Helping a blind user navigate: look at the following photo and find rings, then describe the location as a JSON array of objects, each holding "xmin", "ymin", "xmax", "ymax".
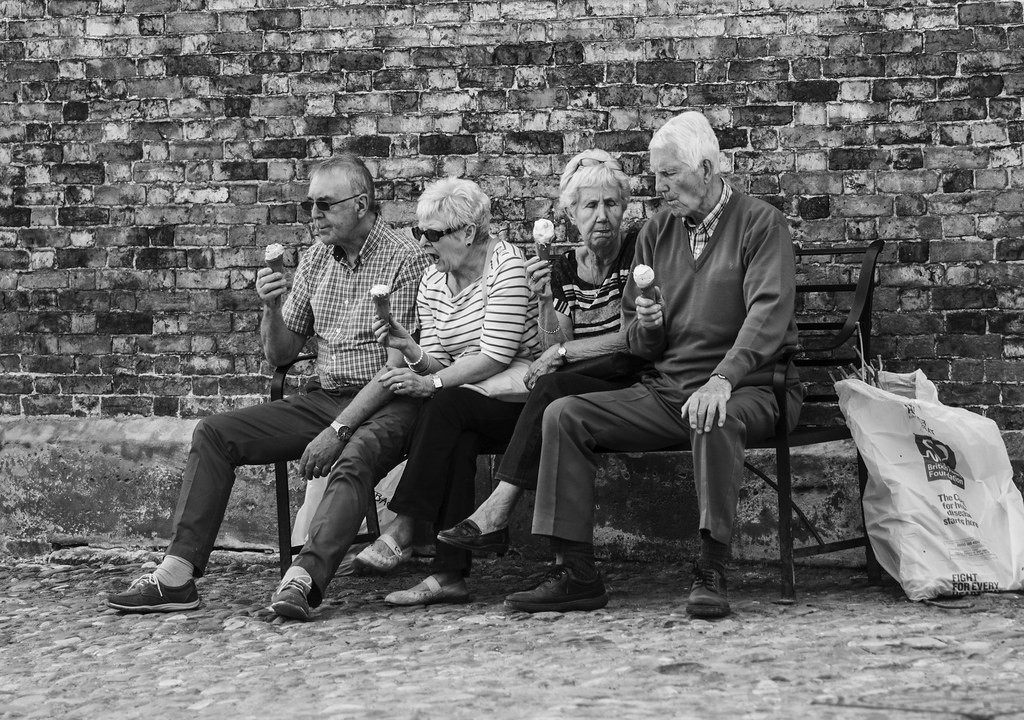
[
  {"xmin": 530, "ymin": 276, "xmax": 538, "ymax": 281},
  {"xmin": 315, "ymin": 466, "xmax": 321, "ymax": 470},
  {"xmin": 397, "ymin": 383, "xmax": 402, "ymax": 390}
]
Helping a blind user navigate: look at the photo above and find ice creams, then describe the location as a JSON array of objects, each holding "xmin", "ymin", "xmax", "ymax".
[
  {"xmin": 265, "ymin": 242, "xmax": 285, "ymax": 305},
  {"xmin": 533, "ymin": 219, "xmax": 554, "ymax": 293},
  {"xmin": 369, "ymin": 285, "xmax": 390, "ymax": 348},
  {"xmin": 633, "ymin": 263, "xmax": 655, "ymax": 305}
]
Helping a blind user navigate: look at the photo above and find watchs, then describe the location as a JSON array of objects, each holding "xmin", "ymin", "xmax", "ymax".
[
  {"xmin": 430, "ymin": 372, "xmax": 443, "ymax": 391},
  {"xmin": 330, "ymin": 421, "xmax": 353, "ymax": 442},
  {"xmin": 558, "ymin": 340, "xmax": 569, "ymax": 366}
]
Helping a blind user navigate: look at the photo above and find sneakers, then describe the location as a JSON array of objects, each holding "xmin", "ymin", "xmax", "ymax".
[
  {"xmin": 271, "ymin": 575, "xmax": 312, "ymax": 618},
  {"xmin": 107, "ymin": 574, "xmax": 200, "ymax": 611},
  {"xmin": 506, "ymin": 564, "xmax": 608, "ymax": 611},
  {"xmin": 687, "ymin": 558, "xmax": 729, "ymax": 615}
]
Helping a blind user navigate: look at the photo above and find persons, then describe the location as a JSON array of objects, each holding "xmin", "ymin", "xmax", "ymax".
[
  {"xmin": 436, "ymin": 148, "xmax": 643, "ymax": 576},
  {"xmin": 352, "ymin": 178, "xmax": 542, "ymax": 606},
  {"xmin": 504, "ymin": 111, "xmax": 809, "ymax": 617},
  {"xmin": 105, "ymin": 155, "xmax": 429, "ymax": 618}
]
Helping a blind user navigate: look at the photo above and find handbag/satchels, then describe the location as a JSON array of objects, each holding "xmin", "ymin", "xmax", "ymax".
[
  {"xmin": 834, "ymin": 368, "xmax": 1024, "ymax": 600},
  {"xmin": 458, "ymin": 357, "xmax": 533, "ymax": 401},
  {"xmin": 290, "ymin": 459, "xmax": 407, "ymax": 549},
  {"xmin": 556, "ymin": 351, "xmax": 643, "ymax": 378}
]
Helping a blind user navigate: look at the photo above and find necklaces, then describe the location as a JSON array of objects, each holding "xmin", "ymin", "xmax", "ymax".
[{"xmin": 589, "ymin": 258, "xmax": 606, "ymax": 296}]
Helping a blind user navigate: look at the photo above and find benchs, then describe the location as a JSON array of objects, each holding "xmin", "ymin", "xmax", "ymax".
[{"xmin": 270, "ymin": 238, "xmax": 884, "ymax": 604}]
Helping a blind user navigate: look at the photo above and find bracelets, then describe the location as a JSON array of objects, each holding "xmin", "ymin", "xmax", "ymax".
[
  {"xmin": 537, "ymin": 317, "xmax": 561, "ymax": 333},
  {"xmin": 403, "ymin": 345, "xmax": 424, "ymax": 366},
  {"xmin": 406, "ymin": 356, "xmax": 432, "ymax": 375},
  {"xmin": 717, "ymin": 373, "xmax": 727, "ymax": 379}
]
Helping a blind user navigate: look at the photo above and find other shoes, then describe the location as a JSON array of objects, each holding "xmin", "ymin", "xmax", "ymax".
[
  {"xmin": 356, "ymin": 536, "xmax": 413, "ymax": 571},
  {"xmin": 437, "ymin": 518, "xmax": 510, "ymax": 552},
  {"xmin": 385, "ymin": 575, "xmax": 469, "ymax": 606}
]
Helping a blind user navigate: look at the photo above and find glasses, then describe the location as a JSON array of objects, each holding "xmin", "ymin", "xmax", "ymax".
[
  {"xmin": 412, "ymin": 224, "xmax": 467, "ymax": 242},
  {"xmin": 575, "ymin": 158, "xmax": 624, "ymax": 173},
  {"xmin": 300, "ymin": 195, "xmax": 359, "ymax": 211}
]
